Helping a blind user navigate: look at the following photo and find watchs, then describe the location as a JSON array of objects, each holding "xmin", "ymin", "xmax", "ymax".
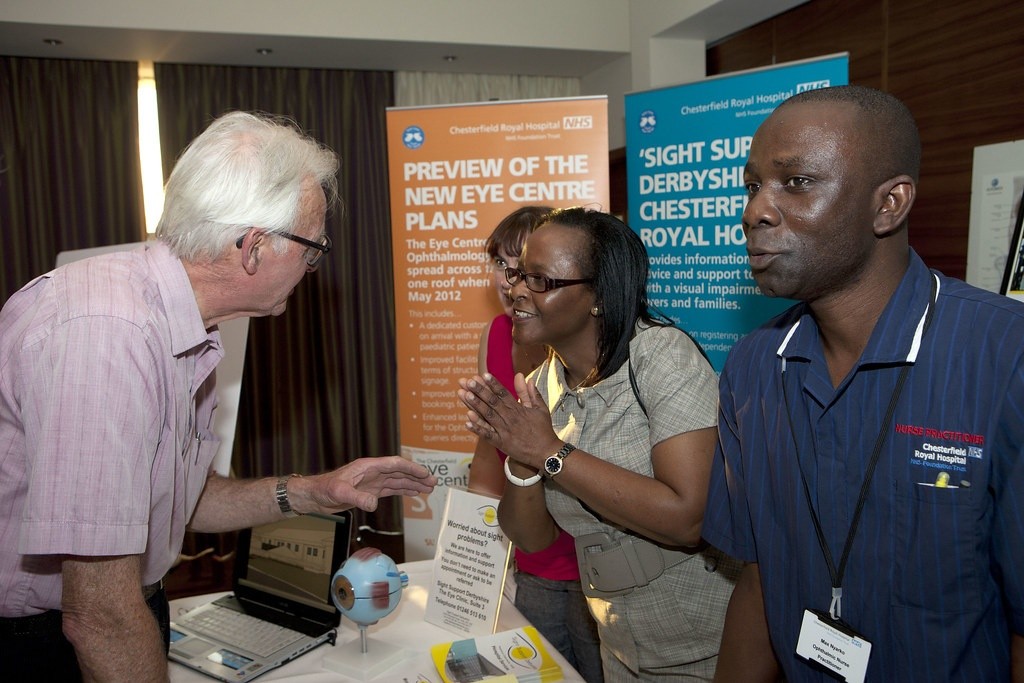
[{"xmin": 543, "ymin": 443, "xmax": 576, "ymax": 481}]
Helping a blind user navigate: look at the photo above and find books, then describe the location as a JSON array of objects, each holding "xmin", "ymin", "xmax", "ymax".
[{"xmin": 431, "ymin": 626, "xmax": 565, "ymax": 682}]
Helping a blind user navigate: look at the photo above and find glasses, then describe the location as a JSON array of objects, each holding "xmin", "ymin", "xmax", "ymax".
[
  {"xmin": 504, "ymin": 266, "xmax": 595, "ymax": 293},
  {"xmin": 236, "ymin": 229, "xmax": 332, "ymax": 267}
]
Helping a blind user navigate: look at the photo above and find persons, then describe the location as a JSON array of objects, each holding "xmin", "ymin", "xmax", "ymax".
[
  {"xmin": 0, "ymin": 110, "xmax": 436, "ymax": 683},
  {"xmin": 459, "ymin": 208, "xmax": 747, "ymax": 683},
  {"xmin": 701, "ymin": 85, "xmax": 1024, "ymax": 683},
  {"xmin": 469, "ymin": 206, "xmax": 604, "ymax": 683}
]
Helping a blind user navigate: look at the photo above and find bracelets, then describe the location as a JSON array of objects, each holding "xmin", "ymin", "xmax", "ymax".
[
  {"xmin": 276, "ymin": 473, "xmax": 302, "ymax": 519},
  {"xmin": 504, "ymin": 456, "xmax": 543, "ymax": 487}
]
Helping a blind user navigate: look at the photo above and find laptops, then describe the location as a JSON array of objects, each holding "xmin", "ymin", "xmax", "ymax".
[{"xmin": 165, "ymin": 508, "xmax": 353, "ymax": 683}]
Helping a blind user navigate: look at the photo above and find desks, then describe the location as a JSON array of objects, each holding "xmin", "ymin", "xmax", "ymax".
[{"xmin": 167, "ymin": 560, "xmax": 588, "ymax": 683}]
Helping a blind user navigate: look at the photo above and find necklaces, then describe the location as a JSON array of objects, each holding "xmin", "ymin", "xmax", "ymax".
[{"xmin": 560, "ymin": 365, "xmax": 595, "ymax": 412}]
